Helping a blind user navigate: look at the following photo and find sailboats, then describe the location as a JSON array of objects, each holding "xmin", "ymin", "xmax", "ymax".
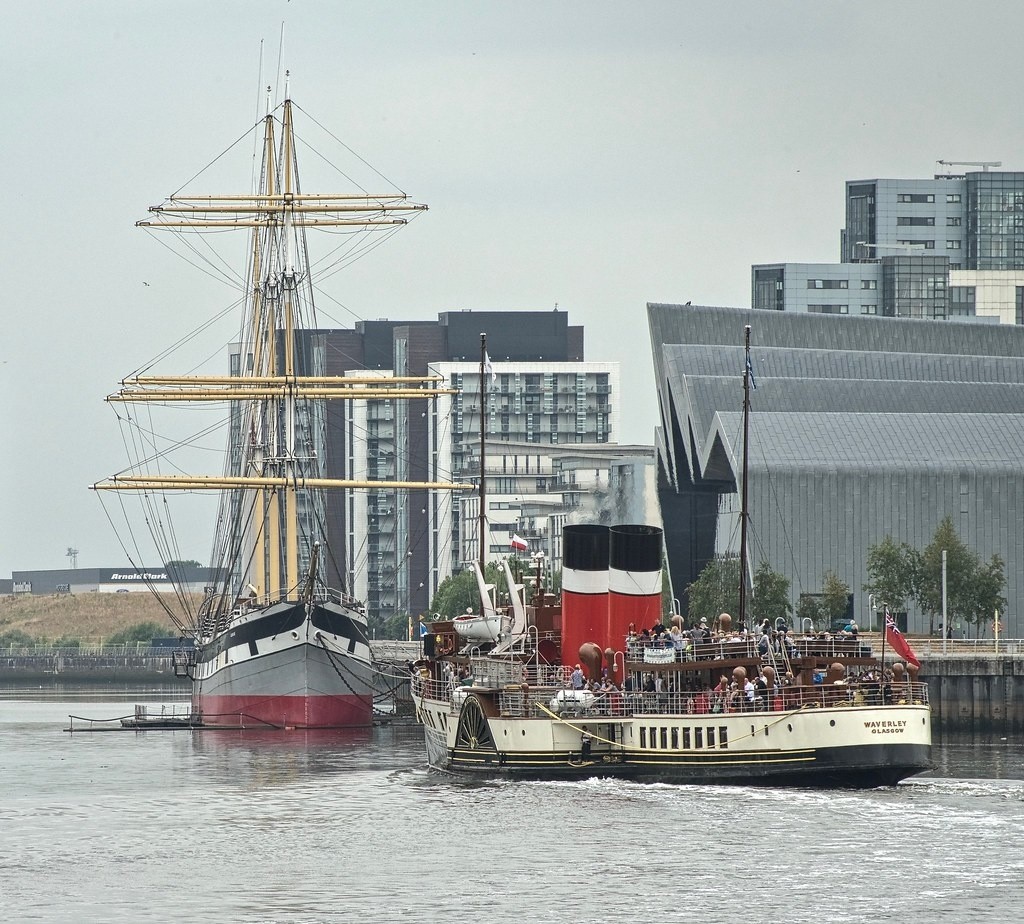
[
  {"xmin": 409, "ymin": 325, "xmax": 933, "ymax": 790},
  {"xmin": 87, "ymin": 21, "xmax": 480, "ymax": 732}
]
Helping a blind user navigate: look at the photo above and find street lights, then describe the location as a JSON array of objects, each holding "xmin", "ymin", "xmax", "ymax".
[
  {"xmin": 519, "ymin": 568, "xmax": 523, "ymax": 606},
  {"xmin": 802, "ymin": 617, "xmax": 814, "ymax": 637},
  {"xmin": 669, "ymin": 598, "xmax": 681, "ymax": 632},
  {"xmin": 869, "ymin": 594, "xmax": 877, "ymax": 631},
  {"xmin": 530, "ymin": 550, "xmax": 545, "ymax": 593}
]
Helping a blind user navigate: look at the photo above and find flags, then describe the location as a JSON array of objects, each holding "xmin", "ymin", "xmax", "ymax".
[{"xmin": 883, "ymin": 607, "xmax": 922, "ymax": 669}]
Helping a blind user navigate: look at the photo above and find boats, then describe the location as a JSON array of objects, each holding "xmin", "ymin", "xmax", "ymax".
[{"xmin": 452, "ymin": 614, "xmax": 512, "ymax": 643}]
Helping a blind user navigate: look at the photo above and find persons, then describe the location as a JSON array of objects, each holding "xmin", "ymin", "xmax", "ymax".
[
  {"xmin": 405, "ymin": 611, "xmax": 895, "ymax": 715},
  {"xmin": 581, "ymin": 725, "xmax": 593, "ymax": 764},
  {"xmin": 512, "ymin": 533, "xmax": 528, "ymax": 550}
]
